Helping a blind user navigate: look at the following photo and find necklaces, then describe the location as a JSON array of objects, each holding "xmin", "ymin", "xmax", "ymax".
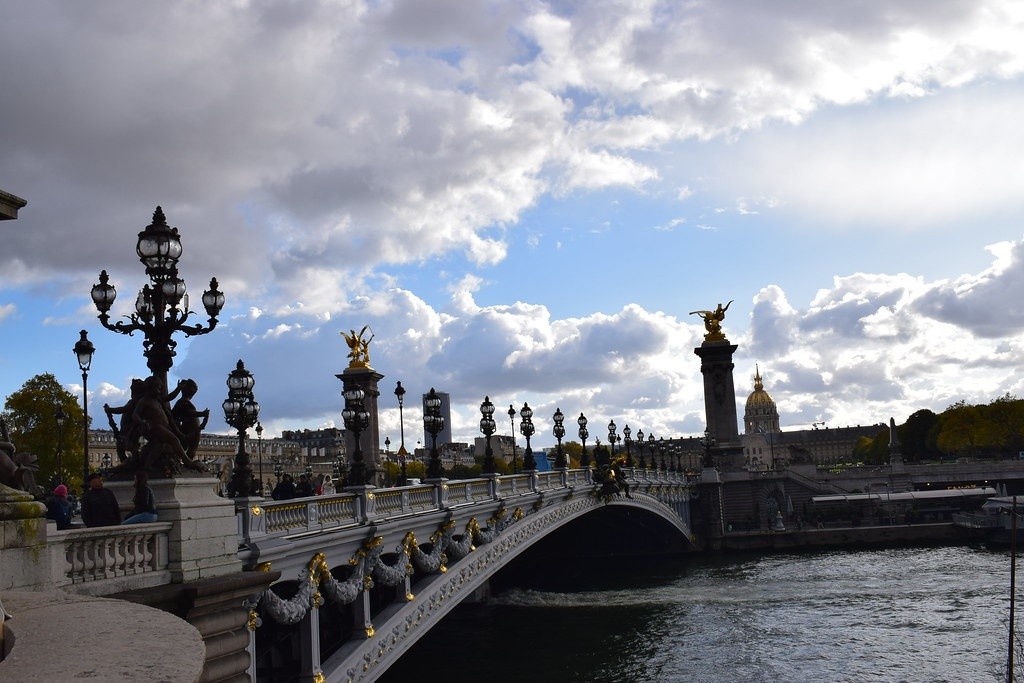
[{"xmin": 321, "ymin": 474, "xmax": 336, "ymax": 496}]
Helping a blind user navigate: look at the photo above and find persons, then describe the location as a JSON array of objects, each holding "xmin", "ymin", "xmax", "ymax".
[
  {"xmin": 378, "ymin": 472, "xmax": 401, "ymax": 488},
  {"xmin": 81, "ymin": 472, "xmax": 121, "ymax": 527},
  {"xmin": 816, "ymin": 515, "xmax": 824, "ymax": 529},
  {"xmin": 132, "ymin": 471, "xmax": 155, "ymax": 507},
  {"xmin": 251, "ymin": 474, "xmax": 315, "ymax": 501},
  {"xmin": 53, "ymin": 485, "xmax": 73, "ymax": 531},
  {"xmin": 599, "ymin": 464, "xmax": 634, "ymax": 499}
]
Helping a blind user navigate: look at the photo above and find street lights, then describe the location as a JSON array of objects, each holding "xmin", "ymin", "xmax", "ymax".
[
  {"xmin": 616, "ymin": 434, "xmax": 621, "ymax": 458},
  {"xmin": 520, "ymin": 401, "xmax": 538, "ymax": 471},
  {"xmin": 221, "ymin": 358, "xmax": 265, "ymax": 499},
  {"xmin": 394, "ymin": 381, "xmax": 407, "ymax": 454},
  {"xmin": 70, "ymin": 330, "xmax": 95, "ymax": 497},
  {"xmin": 422, "ymin": 386, "xmax": 446, "ymax": 479},
  {"xmin": 342, "ymin": 377, "xmax": 372, "ymax": 485},
  {"xmin": 607, "ymin": 420, "xmax": 618, "ymax": 458},
  {"xmin": 274, "ymin": 461, "xmax": 284, "ymax": 485},
  {"xmin": 668, "ymin": 437, "xmax": 676, "ymax": 471},
  {"xmin": 479, "ymin": 395, "xmax": 498, "ymax": 474},
  {"xmin": 417, "ymin": 438, "xmax": 423, "ymax": 462},
  {"xmin": 255, "ymin": 421, "xmax": 264, "ymax": 497},
  {"xmin": 697, "ymin": 426, "xmax": 716, "ymax": 469},
  {"xmin": 385, "ymin": 437, "xmax": 391, "ymax": 485},
  {"xmin": 636, "ymin": 428, "xmax": 647, "ymax": 468},
  {"xmin": 577, "ymin": 412, "xmax": 591, "ymax": 467},
  {"xmin": 688, "ymin": 450, "xmax": 692, "ymax": 470},
  {"xmin": 90, "ymin": 206, "xmax": 225, "ymax": 475},
  {"xmin": 657, "ymin": 436, "xmax": 668, "ymax": 471},
  {"xmin": 507, "ymin": 404, "xmax": 521, "ymax": 474},
  {"xmin": 674, "ymin": 441, "xmax": 683, "ymax": 473},
  {"xmin": 623, "ymin": 424, "xmax": 633, "ymax": 458},
  {"xmin": 552, "ymin": 407, "xmax": 568, "ymax": 470},
  {"xmin": 647, "ymin": 433, "xmax": 658, "ymax": 470}
]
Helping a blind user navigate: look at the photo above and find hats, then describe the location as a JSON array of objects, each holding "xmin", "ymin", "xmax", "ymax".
[{"xmin": 53, "ymin": 485, "xmax": 67, "ymax": 496}]
